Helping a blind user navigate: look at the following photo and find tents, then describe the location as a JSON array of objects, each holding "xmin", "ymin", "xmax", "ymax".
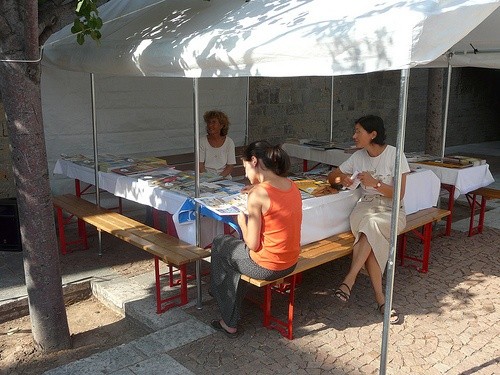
[{"xmin": 41, "ymin": 0, "xmax": 500, "ymax": 375}]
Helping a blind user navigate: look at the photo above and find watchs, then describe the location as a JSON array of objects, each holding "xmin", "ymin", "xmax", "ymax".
[{"xmin": 374, "ymin": 181, "xmax": 381, "ymax": 189}]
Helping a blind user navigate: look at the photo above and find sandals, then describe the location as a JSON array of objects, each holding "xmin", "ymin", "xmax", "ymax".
[
  {"xmin": 378, "ymin": 299, "xmax": 399, "ymax": 324},
  {"xmin": 334, "ymin": 282, "xmax": 353, "ymax": 304}
]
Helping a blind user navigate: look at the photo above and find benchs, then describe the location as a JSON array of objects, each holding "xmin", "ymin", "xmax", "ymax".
[
  {"xmin": 51, "ymin": 192, "xmax": 212, "ymax": 316},
  {"xmin": 239, "ymin": 207, "xmax": 451, "ymax": 339},
  {"xmin": 465, "ymin": 187, "xmax": 500, "ymax": 236}
]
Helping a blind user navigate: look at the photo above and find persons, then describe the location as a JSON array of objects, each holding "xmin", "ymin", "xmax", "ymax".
[
  {"xmin": 208, "ymin": 140, "xmax": 303, "ymax": 339},
  {"xmin": 328, "ymin": 115, "xmax": 411, "ymax": 324},
  {"xmin": 198, "ymin": 111, "xmax": 236, "ymax": 181}
]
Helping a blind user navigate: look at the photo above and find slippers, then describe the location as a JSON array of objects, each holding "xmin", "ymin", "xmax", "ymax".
[{"xmin": 209, "ymin": 318, "xmax": 235, "ymax": 338}]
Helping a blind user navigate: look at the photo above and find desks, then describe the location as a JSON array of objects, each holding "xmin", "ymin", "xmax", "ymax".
[
  {"xmin": 171, "ymin": 165, "xmax": 441, "ymax": 248},
  {"xmin": 52, "ymin": 152, "xmax": 246, "ymax": 251},
  {"xmin": 281, "ymin": 140, "xmax": 495, "ymax": 239}
]
